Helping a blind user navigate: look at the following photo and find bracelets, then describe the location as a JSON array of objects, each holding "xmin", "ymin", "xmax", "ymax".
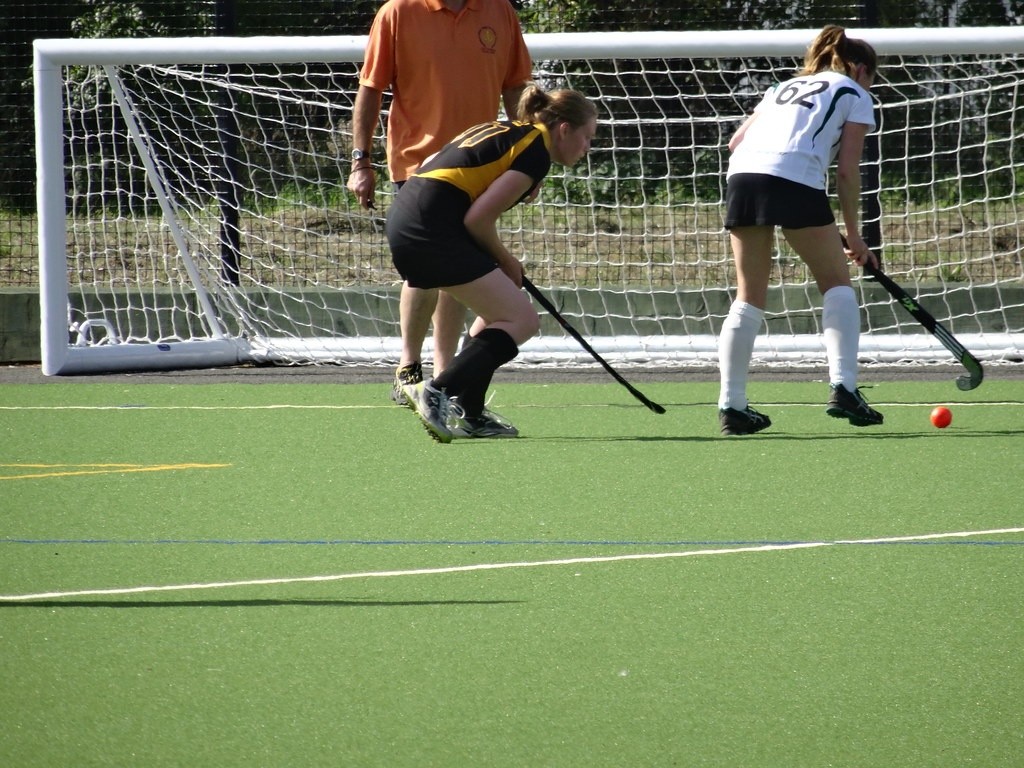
[{"xmin": 349, "ymin": 166, "xmax": 372, "ymax": 174}]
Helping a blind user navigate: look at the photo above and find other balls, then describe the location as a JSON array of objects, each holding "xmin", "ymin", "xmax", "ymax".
[{"xmin": 930, "ymin": 406, "xmax": 953, "ymax": 429}]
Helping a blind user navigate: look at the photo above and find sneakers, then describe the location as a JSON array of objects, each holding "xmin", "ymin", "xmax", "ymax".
[
  {"xmin": 446, "ymin": 396, "xmax": 520, "ymax": 440},
  {"xmin": 719, "ymin": 405, "xmax": 772, "ymax": 436},
  {"xmin": 390, "ymin": 361, "xmax": 424, "ymax": 406},
  {"xmin": 826, "ymin": 383, "xmax": 884, "ymax": 426},
  {"xmin": 398, "ymin": 375, "xmax": 466, "ymax": 444}
]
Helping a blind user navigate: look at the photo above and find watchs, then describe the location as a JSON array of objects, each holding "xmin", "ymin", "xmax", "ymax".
[{"xmin": 351, "ymin": 149, "xmax": 370, "ymax": 160}]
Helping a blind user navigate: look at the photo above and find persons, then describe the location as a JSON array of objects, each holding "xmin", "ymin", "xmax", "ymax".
[
  {"xmin": 346, "ymin": 0, "xmax": 535, "ymax": 404},
  {"xmin": 385, "ymin": 86, "xmax": 597, "ymax": 441},
  {"xmin": 715, "ymin": 24, "xmax": 884, "ymax": 436}
]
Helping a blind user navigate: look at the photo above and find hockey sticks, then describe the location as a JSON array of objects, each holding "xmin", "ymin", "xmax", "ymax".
[
  {"xmin": 521, "ymin": 275, "xmax": 669, "ymax": 416},
  {"xmin": 839, "ymin": 231, "xmax": 987, "ymax": 394}
]
